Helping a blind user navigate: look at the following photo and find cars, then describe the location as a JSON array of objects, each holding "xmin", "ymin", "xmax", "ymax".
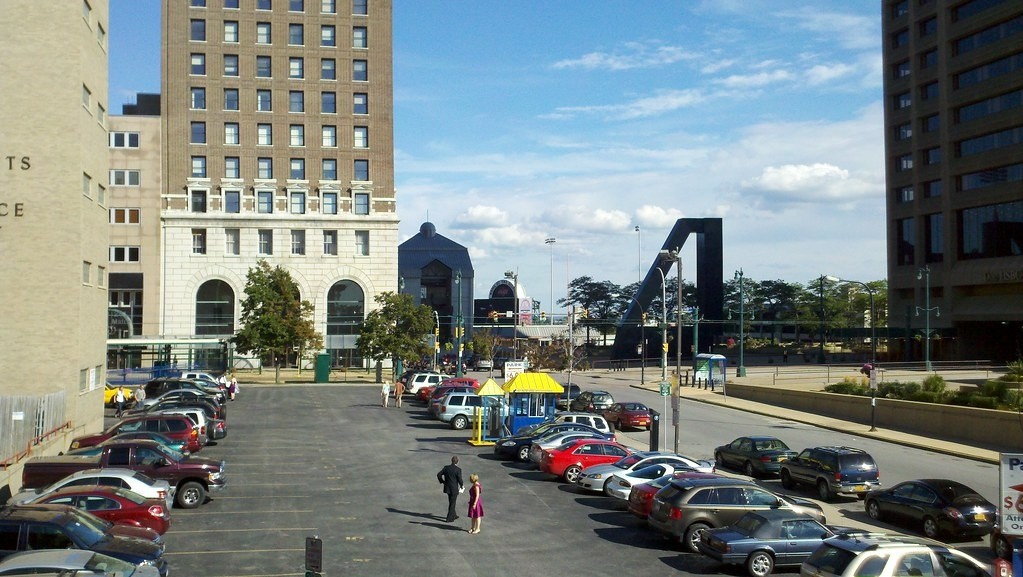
[
  {"xmin": 0, "ymin": 366, "xmax": 230, "ymax": 577},
  {"xmin": 863, "ymin": 478, "xmax": 1000, "ymax": 541},
  {"xmin": 695, "ymin": 509, "xmax": 873, "ymax": 577},
  {"xmin": 574, "ymin": 450, "xmax": 717, "ymax": 498},
  {"xmin": 990, "ymin": 515, "xmax": 1023, "ymax": 565},
  {"xmin": 625, "ymin": 468, "xmax": 762, "ymax": 522},
  {"xmin": 395, "ymin": 359, "xmax": 526, "ymax": 431},
  {"xmin": 605, "ymin": 462, "xmax": 717, "ymax": 504},
  {"xmin": 569, "ymin": 390, "xmax": 616, "ymax": 417},
  {"xmin": 600, "ymin": 401, "xmax": 651, "ymax": 432},
  {"xmin": 799, "ymin": 529, "xmax": 999, "ymax": 577},
  {"xmin": 491, "ymin": 410, "xmax": 653, "ymax": 485},
  {"xmin": 712, "ymin": 434, "xmax": 800, "ymax": 480},
  {"xmin": 554, "ymin": 383, "xmax": 582, "ymax": 410}
]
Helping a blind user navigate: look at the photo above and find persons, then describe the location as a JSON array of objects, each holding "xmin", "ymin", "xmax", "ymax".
[
  {"xmin": 135, "ymin": 385, "xmax": 146, "ymax": 408},
  {"xmin": 231, "ymin": 375, "xmax": 236, "ymax": 401},
  {"xmin": 381, "ymin": 381, "xmax": 390, "ymax": 407},
  {"xmin": 468, "ymin": 474, "xmax": 484, "ymax": 534},
  {"xmin": 394, "ymin": 381, "xmax": 405, "ymax": 407},
  {"xmin": 437, "ymin": 456, "xmax": 464, "ymax": 522},
  {"xmin": 114, "ymin": 386, "xmax": 127, "ymax": 418},
  {"xmin": 860, "ymin": 365, "xmax": 872, "ymax": 378}
]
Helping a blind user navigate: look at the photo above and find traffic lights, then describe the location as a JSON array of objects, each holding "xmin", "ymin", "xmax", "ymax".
[
  {"xmin": 435, "ymin": 327, "xmax": 440, "ymax": 337},
  {"xmin": 541, "ymin": 311, "xmax": 545, "ymax": 321},
  {"xmin": 493, "ymin": 311, "xmax": 498, "ymax": 322}
]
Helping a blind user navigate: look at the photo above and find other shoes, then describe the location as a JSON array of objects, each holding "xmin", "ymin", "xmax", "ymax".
[
  {"xmin": 446, "ymin": 519, "xmax": 454, "ymax": 522},
  {"xmin": 472, "ymin": 529, "xmax": 480, "ymax": 533},
  {"xmin": 454, "ymin": 516, "xmax": 459, "ymax": 519},
  {"xmin": 468, "ymin": 528, "xmax": 475, "ymax": 533}
]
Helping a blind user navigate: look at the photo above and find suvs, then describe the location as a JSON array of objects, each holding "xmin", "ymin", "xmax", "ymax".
[
  {"xmin": 778, "ymin": 444, "xmax": 880, "ymax": 503},
  {"xmin": 646, "ymin": 477, "xmax": 827, "ymax": 555}
]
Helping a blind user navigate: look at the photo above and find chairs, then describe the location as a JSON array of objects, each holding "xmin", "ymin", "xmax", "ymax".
[
  {"xmin": 79, "ymin": 501, "xmax": 87, "ymax": 510},
  {"xmin": 781, "ymin": 526, "xmax": 787, "ymax": 537},
  {"xmin": 763, "ymin": 441, "xmax": 772, "ymax": 448},
  {"xmin": 910, "ymin": 558, "xmax": 930, "ymax": 573},
  {"xmin": 731, "ymin": 495, "xmax": 737, "ymax": 504}
]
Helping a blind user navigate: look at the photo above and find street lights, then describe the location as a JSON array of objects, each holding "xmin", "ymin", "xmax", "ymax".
[
  {"xmin": 913, "ymin": 265, "xmax": 933, "ymax": 372},
  {"xmin": 826, "ymin": 276, "xmax": 877, "ymax": 432},
  {"xmin": 665, "ymin": 255, "xmax": 683, "ymax": 384},
  {"xmin": 634, "ymin": 224, "xmax": 642, "ymax": 286},
  {"xmin": 504, "ymin": 270, "xmax": 517, "ymax": 362},
  {"xmin": 610, "ymin": 293, "xmax": 645, "ymax": 385},
  {"xmin": 394, "ymin": 275, "xmax": 407, "ymax": 379},
  {"xmin": 544, "ymin": 237, "xmax": 557, "ymax": 325},
  {"xmin": 454, "ymin": 270, "xmax": 463, "ymax": 378},
  {"xmin": 733, "ymin": 267, "xmax": 746, "ymax": 378}
]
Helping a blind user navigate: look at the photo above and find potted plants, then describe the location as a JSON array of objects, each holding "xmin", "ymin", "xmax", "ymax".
[{"xmin": 340, "ymin": 359, "xmax": 348, "ymax": 372}]
